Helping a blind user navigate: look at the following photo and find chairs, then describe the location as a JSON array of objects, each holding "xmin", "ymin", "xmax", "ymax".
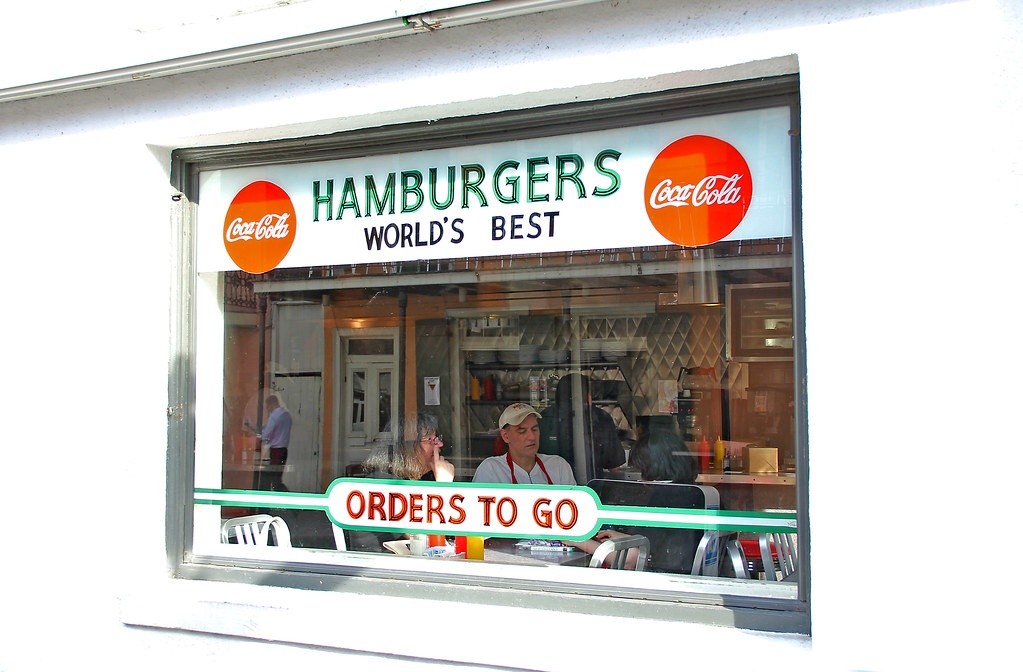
[
  {"xmin": 330, "ymin": 520, "xmax": 350, "ymax": 552},
  {"xmin": 726, "ymin": 539, "xmax": 753, "ymax": 581},
  {"xmin": 222, "ymin": 514, "xmax": 282, "ymax": 546},
  {"xmin": 688, "ymin": 529, "xmax": 742, "ymax": 577},
  {"xmin": 759, "ymin": 529, "xmax": 797, "ymax": 585},
  {"xmin": 589, "ymin": 534, "xmax": 651, "ymax": 573}
]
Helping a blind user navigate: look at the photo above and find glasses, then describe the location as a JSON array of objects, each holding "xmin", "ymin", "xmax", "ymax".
[{"xmin": 417, "ymin": 435, "xmax": 443, "ymax": 445}]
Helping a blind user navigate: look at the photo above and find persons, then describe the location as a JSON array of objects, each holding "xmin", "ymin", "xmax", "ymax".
[
  {"xmin": 536, "ymin": 372, "xmax": 628, "ymax": 482},
  {"xmin": 356, "ymin": 410, "xmax": 456, "ymax": 540},
  {"xmin": 256, "ymin": 394, "xmax": 302, "ymax": 517},
  {"xmin": 470, "ymin": 402, "xmax": 578, "ymax": 486},
  {"xmin": 560, "ymin": 428, "xmax": 700, "ymax": 575}
]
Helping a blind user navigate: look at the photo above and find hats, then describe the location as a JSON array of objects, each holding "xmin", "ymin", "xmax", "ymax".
[{"xmin": 499, "ymin": 402, "xmax": 542, "ymax": 430}]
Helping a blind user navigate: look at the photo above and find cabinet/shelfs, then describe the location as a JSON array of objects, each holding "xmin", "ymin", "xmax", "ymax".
[{"xmin": 454, "ymin": 313, "xmax": 695, "ymax": 479}]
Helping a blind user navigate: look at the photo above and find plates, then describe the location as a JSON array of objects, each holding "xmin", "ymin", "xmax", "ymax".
[{"xmin": 460, "ymin": 334, "xmax": 628, "ymax": 366}]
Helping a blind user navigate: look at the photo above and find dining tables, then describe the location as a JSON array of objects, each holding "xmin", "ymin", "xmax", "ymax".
[{"xmin": 368, "ymin": 537, "xmax": 594, "ymax": 568}]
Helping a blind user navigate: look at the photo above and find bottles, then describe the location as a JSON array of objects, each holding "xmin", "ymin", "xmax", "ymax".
[
  {"xmin": 471, "ymin": 375, "xmax": 480, "ymax": 400},
  {"xmin": 713, "ymin": 435, "xmax": 726, "ymax": 472},
  {"xmin": 483, "ymin": 373, "xmax": 494, "ymax": 401},
  {"xmin": 698, "ymin": 434, "xmax": 711, "ymax": 471},
  {"xmin": 722, "ymin": 452, "xmax": 742, "ymax": 473},
  {"xmin": 496, "ymin": 379, "xmax": 502, "ymax": 400},
  {"xmin": 235, "ymin": 438, "xmax": 270, "ymax": 463}
]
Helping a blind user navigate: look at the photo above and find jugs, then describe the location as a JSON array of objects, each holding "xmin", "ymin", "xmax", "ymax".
[{"xmin": 677, "ymin": 365, "xmax": 714, "ymax": 390}]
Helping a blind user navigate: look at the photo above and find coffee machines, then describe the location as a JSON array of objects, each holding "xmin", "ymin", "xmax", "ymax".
[{"xmin": 677, "ymin": 388, "xmax": 726, "ymax": 442}]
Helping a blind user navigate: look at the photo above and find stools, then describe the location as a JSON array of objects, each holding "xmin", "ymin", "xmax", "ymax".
[{"xmin": 733, "ymin": 539, "xmax": 794, "ymax": 581}]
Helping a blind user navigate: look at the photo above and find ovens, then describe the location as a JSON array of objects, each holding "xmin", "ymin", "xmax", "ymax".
[{"xmin": 724, "ymin": 284, "xmax": 794, "ymax": 361}]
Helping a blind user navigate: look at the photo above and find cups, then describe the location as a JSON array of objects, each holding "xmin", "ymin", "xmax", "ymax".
[
  {"xmin": 467, "ymin": 536, "xmax": 484, "ymax": 560},
  {"xmin": 428, "ymin": 534, "xmax": 444, "ymax": 548},
  {"xmin": 409, "ymin": 534, "xmax": 427, "ymax": 556},
  {"xmin": 454, "ymin": 535, "xmax": 467, "ymax": 560}
]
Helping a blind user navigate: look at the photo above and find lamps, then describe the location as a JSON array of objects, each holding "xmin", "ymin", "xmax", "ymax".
[{"xmin": 678, "ymin": 249, "xmax": 721, "ymax": 307}]
